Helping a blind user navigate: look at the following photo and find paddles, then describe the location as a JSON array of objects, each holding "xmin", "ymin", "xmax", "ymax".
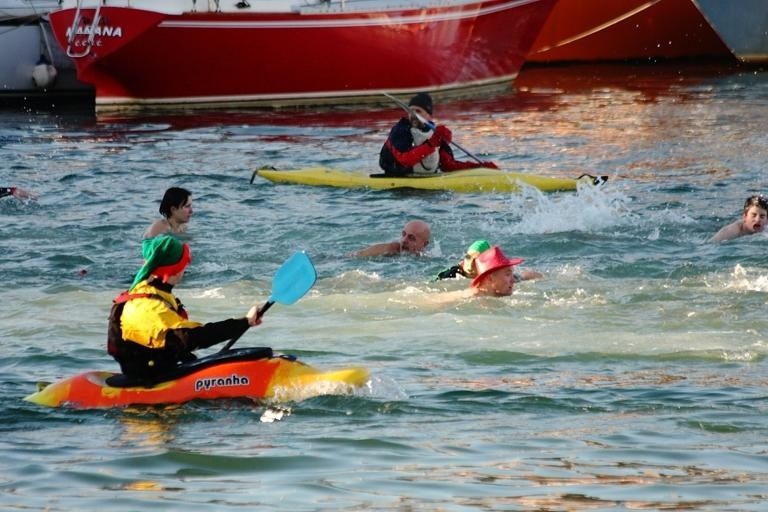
[
  {"xmin": 217, "ymin": 251, "xmax": 316, "ymax": 352},
  {"xmin": 383, "ymin": 92, "xmax": 485, "ymax": 164}
]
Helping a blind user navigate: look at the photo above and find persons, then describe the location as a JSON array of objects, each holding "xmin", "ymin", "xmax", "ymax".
[
  {"xmin": 0, "ymin": 187, "xmax": 38, "ymax": 205},
  {"xmin": 355, "ymin": 220, "xmax": 431, "ymax": 257},
  {"xmin": 430, "ymin": 240, "xmax": 542, "ymax": 282},
  {"xmin": 143, "ymin": 187, "xmax": 193, "ymax": 239},
  {"xmin": 470, "ymin": 245, "xmax": 525, "ymax": 297},
  {"xmin": 707, "ymin": 196, "xmax": 768, "ymax": 243},
  {"xmin": 107, "ymin": 233, "xmax": 263, "ymax": 389},
  {"xmin": 379, "ymin": 93, "xmax": 497, "ymax": 178}
]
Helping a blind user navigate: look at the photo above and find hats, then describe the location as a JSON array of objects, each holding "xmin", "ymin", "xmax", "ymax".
[
  {"xmin": 128, "ymin": 233, "xmax": 188, "ymax": 291},
  {"xmin": 408, "ymin": 93, "xmax": 432, "ymax": 115},
  {"xmin": 468, "ymin": 240, "xmax": 523, "ymax": 287}
]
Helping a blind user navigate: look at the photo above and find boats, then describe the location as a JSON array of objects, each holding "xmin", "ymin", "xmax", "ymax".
[
  {"xmin": 252, "ymin": 158, "xmax": 614, "ymax": 200},
  {"xmin": 0, "ymin": 0, "xmax": 104, "ymax": 100},
  {"xmin": 22, "ymin": 347, "xmax": 370, "ymax": 418},
  {"xmin": 519, "ymin": 0, "xmax": 768, "ymax": 64},
  {"xmin": 43, "ymin": 0, "xmax": 561, "ymax": 124}
]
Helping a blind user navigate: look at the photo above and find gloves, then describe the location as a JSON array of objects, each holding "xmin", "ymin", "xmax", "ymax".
[{"xmin": 429, "ymin": 124, "xmax": 451, "ymax": 146}]
